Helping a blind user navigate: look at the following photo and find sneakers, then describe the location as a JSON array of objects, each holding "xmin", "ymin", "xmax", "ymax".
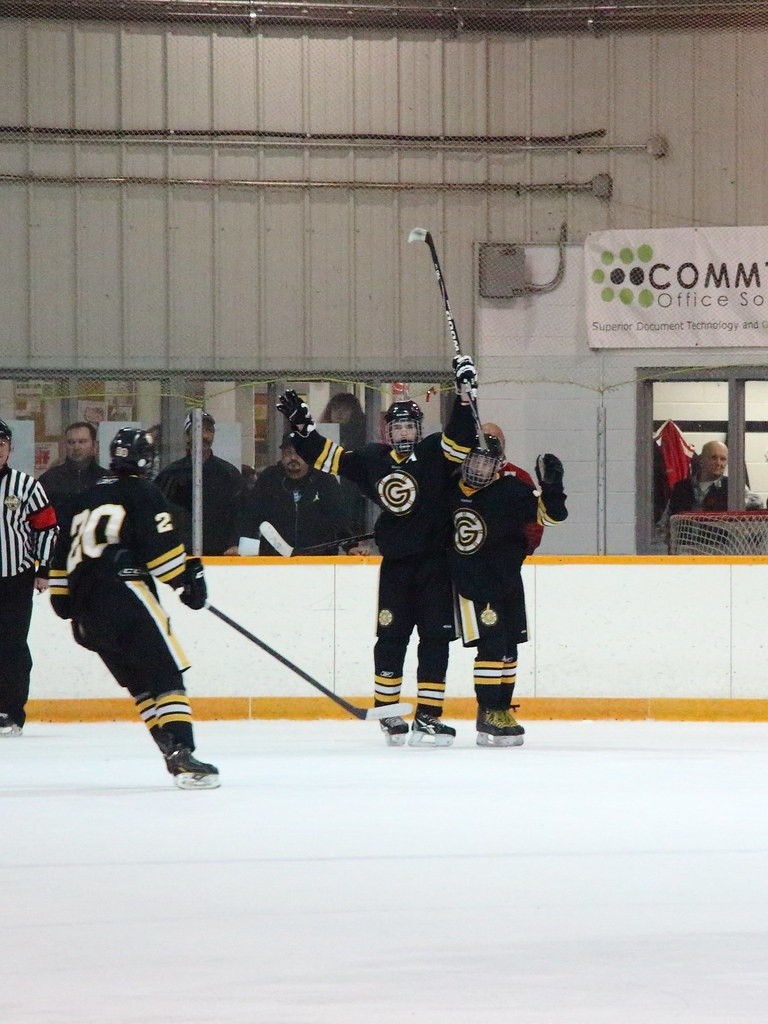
[
  {"xmin": 158, "ymin": 734, "xmax": 225, "ymax": 790},
  {"xmin": 408, "ymin": 705, "xmax": 456, "ymax": 746},
  {"xmin": 381, "ymin": 717, "xmax": 409, "ymax": 747},
  {"xmin": 476, "ymin": 704, "xmax": 524, "ymax": 748},
  {"xmin": 0, "ymin": 711, "xmax": 22, "ymax": 737}
]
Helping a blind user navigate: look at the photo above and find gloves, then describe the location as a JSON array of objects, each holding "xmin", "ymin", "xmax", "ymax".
[
  {"xmin": 48, "ymin": 569, "xmax": 73, "ymax": 619},
  {"xmin": 536, "ymin": 452, "xmax": 564, "ymax": 489},
  {"xmin": 169, "ymin": 557, "xmax": 207, "ymax": 610},
  {"xmin": 452, "ymin": 356, "xmax": 478, "ymax": 399},
  {"xmin": 276, "ymin": 391, "xmax": 315, "ymax": 437}
]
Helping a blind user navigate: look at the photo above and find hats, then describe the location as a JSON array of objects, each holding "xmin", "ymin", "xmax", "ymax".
[
  {"xmin": 280, "ymin": 431, "xmax": 294, "ymax": 450},
  {"xmin": 183, "ymin": 410, "xmax": 215, "ymax": 433}
]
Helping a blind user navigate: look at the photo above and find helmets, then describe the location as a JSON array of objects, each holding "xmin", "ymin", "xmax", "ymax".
[
  {"xmin": 383, "ymin": 400, "xmax": 423, "ymax": 452},
  {"xmin": 0, "ymin": 419, "xmax": 12, "ymax": 439},
  {"xmin": 461, "ymin": 434, "xmax": 504, "ymax": 489},
  {"xmin": 110, "ymin": 427, "xmax": 160, "ymax": 481}
]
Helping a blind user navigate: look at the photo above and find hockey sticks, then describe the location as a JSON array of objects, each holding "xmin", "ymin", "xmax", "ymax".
[
  {"xmin": 258, "ymin": 520, "xmax": 376, "ymax": 558},
  {"xmin": 176, "ymin": 583, "xmax": 414, "ymax": 722},
  {"xmin": 406, "ymin": 225, "xmax": 491, "ymax": 455}
]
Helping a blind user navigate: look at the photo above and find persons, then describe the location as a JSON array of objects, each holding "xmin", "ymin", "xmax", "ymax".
[
  {"xmin": 670, "ymin": 441, "xmax": 728, "ymax": 544},
  {"xmin": 481, "ymin": 423, "xmax": 544, "ymax": 556},
  {"xmin": 153, "ymin": 410, "xmax": 242, "ymax": 556},
  {"xmin": 317, "ymin": 393, "xmax": 367, "ymax": 542},
  {"xmin": 223, "ymin": 433, "xmax": 371, "ymax": 557},
  {"xmin": 451, "ymin": 434, "xmax": 568, "ymax": 747},
  {"xmin": 38, "ymin": 422, "xmax": 109, "ymax": 511},
  {"xmin": 0, "ymin": 419, "xmax": 60, "ymax": 737},
  {"xmin": 277, "ymin": 354, "xmax": 481, "ymax": 747},
  {"xmin": 49, "ymin": 427, "xmax": 221, "ymax": 790}
]
